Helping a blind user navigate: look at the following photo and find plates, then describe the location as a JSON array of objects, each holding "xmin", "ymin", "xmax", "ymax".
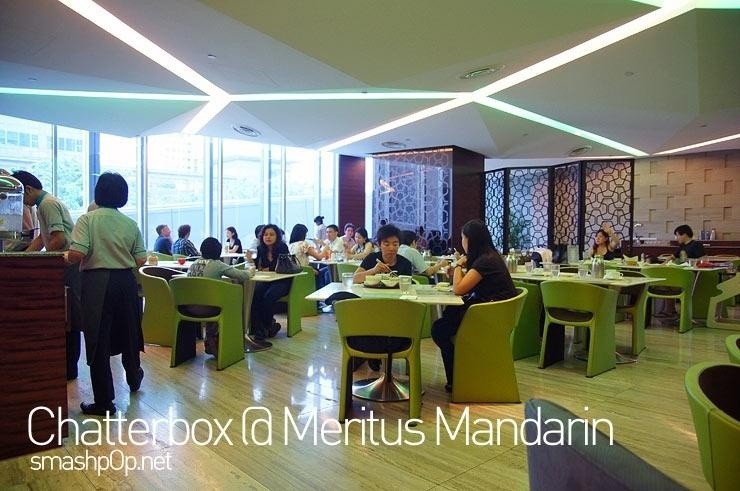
[
  {"xmin": 415, "ymin": 282, "xmax": 451, "ymax": 296},
  {"xmin": 605, "ymin": 276, "xmax": 623, "ymax": 280}
]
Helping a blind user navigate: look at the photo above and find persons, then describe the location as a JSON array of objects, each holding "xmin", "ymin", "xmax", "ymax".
[
  {"xmin": 63, "ymin": 172, "xmax": 147, "ymax": 417},
  {"xmin": 588, "ymin": 222, "xmax": 620, "ymax": 260},
  {"xmin": 431, "ymin": 220, "xmax": 516, "ymax": 393},
  {"xmin": 656, "ymin": 225, "xmax": 707, "ymax": 319},
  {"xmin": 4, "ymin": 172, "xmax": 450, "ymax": 381}
]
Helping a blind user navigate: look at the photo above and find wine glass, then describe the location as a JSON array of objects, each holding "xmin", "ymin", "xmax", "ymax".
[{"xmin": 178, "ymin": 259, "xmax": 186, "ymax": 271}]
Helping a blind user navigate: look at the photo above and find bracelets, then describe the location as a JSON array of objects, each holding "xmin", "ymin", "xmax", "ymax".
[{"xmin": 453, "ymin": 264, "xmax": 463, "ymax": 271}]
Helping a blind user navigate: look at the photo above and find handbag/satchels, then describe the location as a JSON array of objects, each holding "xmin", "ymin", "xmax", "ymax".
[{"xmin": 275, "ymin": 254, "xmax": 303, "ymax": 274}]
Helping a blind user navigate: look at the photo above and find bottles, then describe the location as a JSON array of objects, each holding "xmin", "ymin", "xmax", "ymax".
[
  {"xmin": 149, "ymin": 253, "xmax": 158, "ymax": 266},
  {"xmin": 592, "ymin": 256, "xmax": 604, "ymax": 279},
  {"xmin": 506, "ymin": 253, "xmax": 516, "ymax": 274}
]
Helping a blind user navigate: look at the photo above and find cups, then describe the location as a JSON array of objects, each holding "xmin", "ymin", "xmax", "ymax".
[
  {"xmin": 578, "ymin": 266, "xmax": 588, "ymax": 280},
  {"xmin": 342, "ymin": 273, "xmax": 354, "ymax": 291},
  {"xmin": 398, "ymin": 276, "xmax": 412, "ymax": 295},
  {"xmin": 524, "ymin": 263, "xmax": 533, "ymax": 276},
  {"xmin": 605, "ymin": 270, "xmax": 616, "ymax": 280},
  {"xmin": 551, "ymin": 265, "xmax": 560, "ymax": 279}
]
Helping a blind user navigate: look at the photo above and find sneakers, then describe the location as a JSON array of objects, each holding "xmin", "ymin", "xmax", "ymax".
[
  {"xmin": 129, "ymin": 367, "xmax": 144, "ymax": 391},
  {"xmin": 80, "ymin": 401, "xmax": 117, "ymax": 416}
]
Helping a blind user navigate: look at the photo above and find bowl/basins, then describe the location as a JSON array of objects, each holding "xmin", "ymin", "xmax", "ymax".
[
  {"xmin": 381, "ymin": 280, "xmax": 399, "ymax": 289},
  {"xmin": 365, "ymin": 276, "xmax": 382, "ymax": 286}
]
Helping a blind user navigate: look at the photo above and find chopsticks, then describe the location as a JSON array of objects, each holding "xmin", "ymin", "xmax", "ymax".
[{"xmin": 376, "ymin": 258, "xmax": 398, "ymax": 278}]
